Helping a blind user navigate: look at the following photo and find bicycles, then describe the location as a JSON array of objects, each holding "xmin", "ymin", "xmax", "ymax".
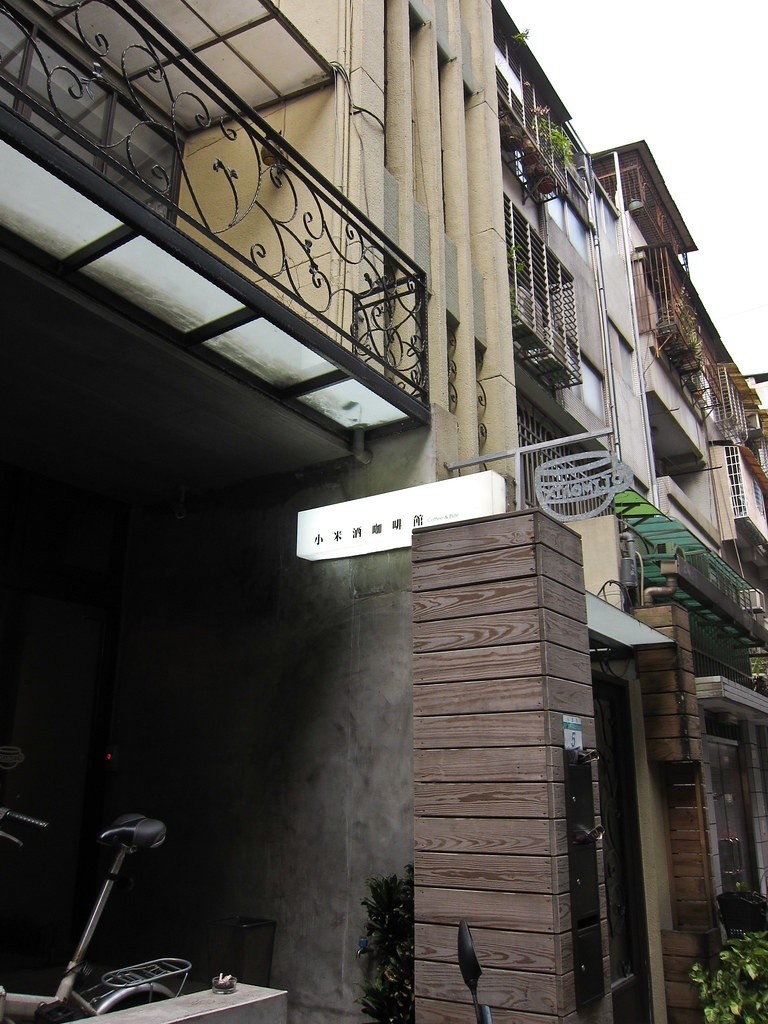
[{"xmin": 0, "ymin": 800, "xmax": 195, "ymax": 1024}]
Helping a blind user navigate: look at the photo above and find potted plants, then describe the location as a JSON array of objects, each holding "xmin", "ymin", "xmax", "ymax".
[
  {"xmin": 500, "ymin": 100, "xmax": 575, "ymax": 194},
  {"xmin": 260, "ymin": 128, "xmax": 282, "ymax": 165}
]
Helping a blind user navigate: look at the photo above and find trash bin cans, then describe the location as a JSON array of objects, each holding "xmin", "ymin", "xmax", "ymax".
[
  {"xmin": 717, "ymin": 892, "xmax": 768, "ymax": 938},
  {"xmin": 205, "ymin": 915, "xmax": 277, "ymax": 987}
]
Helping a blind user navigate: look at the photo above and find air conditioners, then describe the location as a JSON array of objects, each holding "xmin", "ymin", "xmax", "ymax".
[
  {"xmin": 513, "ymin": 286, "xmax": 545, "ymax": 350},
  {"xmin": 745, "ymin": 410, "xmax": 764, "ymax": 439},
  {"xmin": 739, "ymin": 588, "xmax": 765, "ymax": 613}
]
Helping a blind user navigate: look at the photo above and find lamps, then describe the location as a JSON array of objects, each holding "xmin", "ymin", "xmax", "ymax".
[
  {"xmin": 572, "ymin": 748, "xmax": 600, "ymax": 765},
  {"xmin": 579, "ymin": 825, "xmax": 605, "ymax": 844},
  {"xmin": 627, "ymin": 197, "xmax": 645, "ymax": 213}
]
